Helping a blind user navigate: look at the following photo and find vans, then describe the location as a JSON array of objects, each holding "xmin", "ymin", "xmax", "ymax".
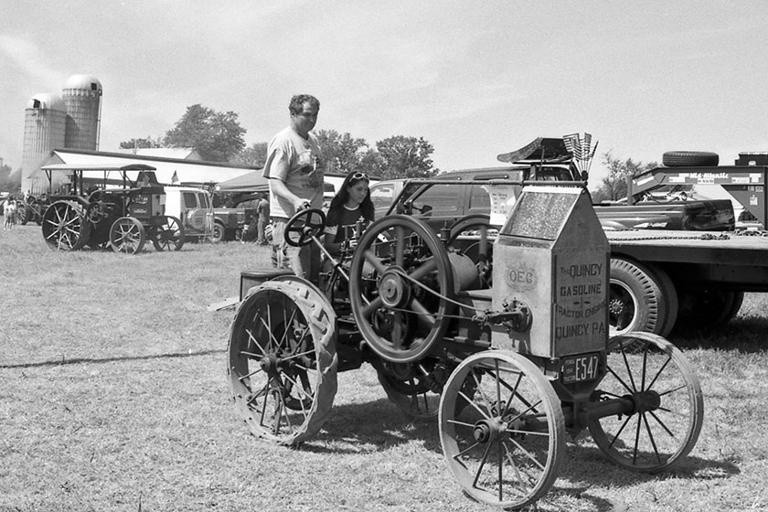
[{"xmin": 162, "ymin": 185, "xmax": 214, "ymax": 244}]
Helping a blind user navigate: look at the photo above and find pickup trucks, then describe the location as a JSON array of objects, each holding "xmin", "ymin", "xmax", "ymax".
[{"xmin": 207, "ymin": 207, "xmax": 257, "ymax": 244}]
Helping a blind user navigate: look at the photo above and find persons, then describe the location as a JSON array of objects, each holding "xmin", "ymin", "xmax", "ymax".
[
  {"xmin": 256, "ymin": 193, "xmax": 269, "ymax": 245},
  {"xmin": 240, "ymin": 220, "xmax": 251, "ymax": 244},
  {"xmin": 262, "ymin": 92, "xmax": 324, "ymax": 280},
  {"xmin": 3, "ymin": 196, "xmax": 19, "ymax": 231},
  {"xmin": 322, "ymin": 168, "xmax": 378, "ymax": 256}
]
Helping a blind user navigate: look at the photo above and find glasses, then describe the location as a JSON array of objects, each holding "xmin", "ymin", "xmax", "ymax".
[{"xmin": 350, "ymin": 172, "xmax": 371, "ymax": 181}]
